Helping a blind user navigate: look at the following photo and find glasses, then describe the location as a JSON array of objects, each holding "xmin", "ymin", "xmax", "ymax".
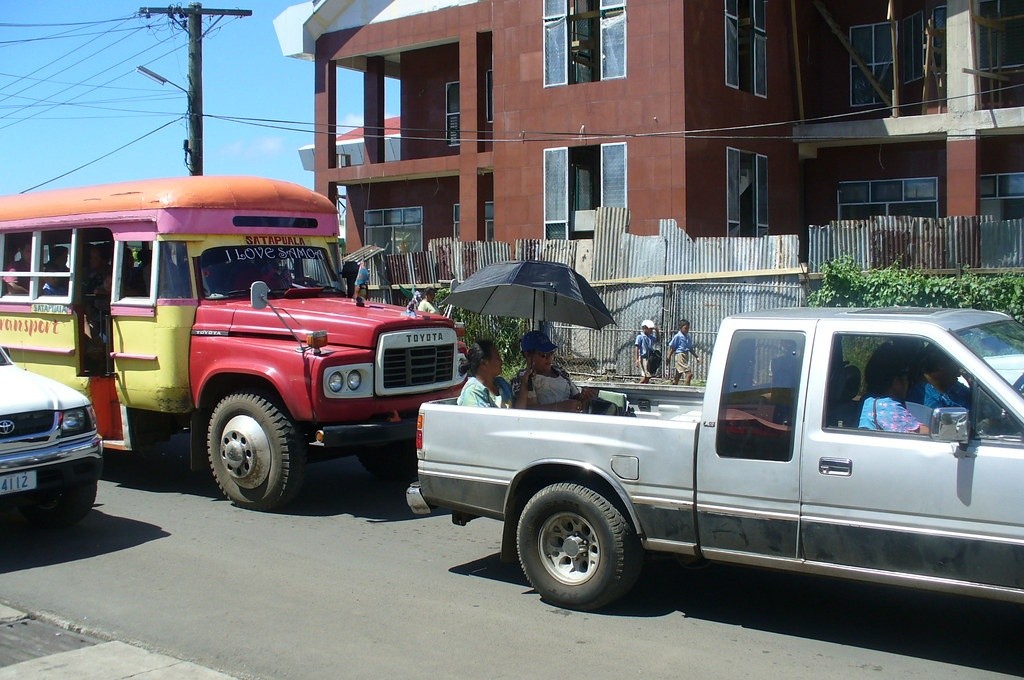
[{"xmin": 536, "ymin": 351, "xmax": 555, "ymax": 358}]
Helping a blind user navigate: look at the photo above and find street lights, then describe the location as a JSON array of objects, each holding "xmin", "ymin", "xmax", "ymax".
[{"xmin": 136, "ymin": 65, "xmax": 205, "ymax": 176}]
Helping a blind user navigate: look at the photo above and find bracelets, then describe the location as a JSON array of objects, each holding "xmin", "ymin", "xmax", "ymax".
[{"xmin": 555, "ymin": 401, "xmax": 557, "ymax": 411}]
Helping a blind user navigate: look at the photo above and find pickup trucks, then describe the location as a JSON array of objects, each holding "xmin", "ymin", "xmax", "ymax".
[{"xmin": 405, "ymin": 307, "xmax": 1024, "ymax": 613}]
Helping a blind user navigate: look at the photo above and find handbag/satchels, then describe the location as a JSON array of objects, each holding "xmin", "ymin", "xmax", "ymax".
[{"xmin": 646, "ymin": 350, "xmax": 662, "ymax": 373}]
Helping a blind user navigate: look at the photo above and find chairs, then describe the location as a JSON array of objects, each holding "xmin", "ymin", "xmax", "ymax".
[
  {"xmin": 829, "ymin": 365, "xmax": 861, "ymax": 400},
  {"xmin": 200, "ymin": 262, "xmax": 229, "ymax": 293}
]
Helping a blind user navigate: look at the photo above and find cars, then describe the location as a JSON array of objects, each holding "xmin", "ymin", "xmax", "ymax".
[{"xmin": 0, "ymin": 345, "xmax": 104, "ymax": 530}]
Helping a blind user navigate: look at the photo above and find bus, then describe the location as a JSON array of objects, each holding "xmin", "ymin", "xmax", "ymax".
[{"xmin": 1, "ymin": 174, "xmax": 470, "ymax": 512}]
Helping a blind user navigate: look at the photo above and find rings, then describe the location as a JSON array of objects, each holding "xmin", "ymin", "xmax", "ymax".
[{"xmin": 576, "ymin": 406, "xmax": 580, "ymax": 410}]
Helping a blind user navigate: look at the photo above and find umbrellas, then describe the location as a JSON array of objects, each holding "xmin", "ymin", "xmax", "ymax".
[{"xmin": 441, "ymin": 260, "xmax": 617, "ymax": 332}]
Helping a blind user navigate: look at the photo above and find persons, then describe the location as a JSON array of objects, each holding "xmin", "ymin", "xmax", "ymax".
[
  {"xmin": 635, "ymin": 319, "xmax": 700, "ymax": 386},
  {"xmin": 2, "ymin": 238, "xmax": 295, "ymax": 378},
  {"xmin": 457, "ymin": 331, "xmax": 599, "ymax": 413},
  {"xmin": 855, "ymin": 342, "xmax": 994, "ymax": 438},
  {"xmin": 418, "ymin": 286, "xmax": 440, "ymax": 315}
]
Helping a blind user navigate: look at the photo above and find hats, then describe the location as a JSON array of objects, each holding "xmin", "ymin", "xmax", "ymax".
[
  {"xmin": 521, "ymin": 331, "xmax": 558, "ymax": 352},
  {"xmin": 642, "ymin": 319, "xmax": 654, "ymax": 328}
]
